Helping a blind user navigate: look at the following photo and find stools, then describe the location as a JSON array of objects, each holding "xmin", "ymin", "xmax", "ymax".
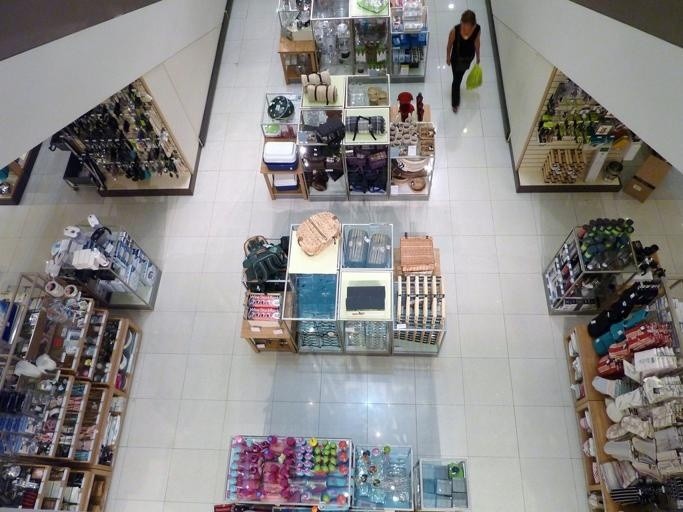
[{"xmin": 63, "ymin": 152, "xmax": 99, "ymax": 191}]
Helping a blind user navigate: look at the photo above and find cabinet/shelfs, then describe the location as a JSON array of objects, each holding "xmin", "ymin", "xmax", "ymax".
[
  {"xmin": 57, "ymin": 222, "xmax": 161, "ymax": 311},
  {"xmin": 260, "ymin": 71, "xmax": 436, "ymax": 202},
  {"xmin": 542, "ymin": 225, "xmax": 681, "ymax": 512},
  {"xmin": 275, "ymin": 0, "xmax": 428, "ymax": 85},
  {"xmin": 1, "ymin": 269, "xmax": 143, "ymax": 510},
  {"xmin": 239, "ymin": 222, "xmax": 447, "ymax": 357}
]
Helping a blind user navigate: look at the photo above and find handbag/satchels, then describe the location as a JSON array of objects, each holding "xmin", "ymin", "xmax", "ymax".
[
  {"xmin": 301, "ymin": 70, "xmax": 331, "ymax": 93},
  {"xmin": 296, "ymin": 213, "xmax": 340, "ymax": 256},
  {"xmin": 348, "ymin": 116, "xmax": 384, "ymax": 134},
  {"xmin": 305, "ymin": 84, "xmax": 337, "ymax": 103},
  {"xmin": 345, "ymin": 147, "xmax": 387, "ymax": 193},
  {"xmin": 317, "ymin": 120, "xmax": 345, "ymax": 142},
  {"xmin": 304, "ymin": 146, "xmax": 344, "ymax": 191}
]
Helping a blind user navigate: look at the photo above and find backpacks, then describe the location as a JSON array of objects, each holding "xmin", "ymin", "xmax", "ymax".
[{"xmin": 243, "ymin": 244, "xmax": 287, "ymax": 283}]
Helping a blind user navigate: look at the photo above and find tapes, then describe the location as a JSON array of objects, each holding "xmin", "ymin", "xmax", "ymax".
[
  {"xmin": 44, "ymin": 281, "xmax": 77, "ymax": 300},
  {"xmin": 390, "ymin": 124, "xmax": 418, "ymax": 147}
]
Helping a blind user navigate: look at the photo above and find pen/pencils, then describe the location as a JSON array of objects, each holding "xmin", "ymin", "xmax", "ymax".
[{"xmin": 56, "ymin": 383, "xmax": 102, "ymax": 461}]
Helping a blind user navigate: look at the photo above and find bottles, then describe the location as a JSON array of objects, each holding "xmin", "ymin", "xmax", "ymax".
[
  {"xmin": 355, "ymin": 40, "xmax": 386, "ymax": 69},
  {"xmin": 622, "ymin": 280, "xmax": 662, "ymax": 305}
]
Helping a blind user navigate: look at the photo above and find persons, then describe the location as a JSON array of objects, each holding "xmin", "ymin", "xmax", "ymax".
[{"xmin": 447, "ymin": 9, "xmax": 481, "ymax": 113}]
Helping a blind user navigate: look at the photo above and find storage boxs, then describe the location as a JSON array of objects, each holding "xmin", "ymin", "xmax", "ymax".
[{"xmin": 624, "ymin": 153, "xmax": 671, "ymax": 204}]
